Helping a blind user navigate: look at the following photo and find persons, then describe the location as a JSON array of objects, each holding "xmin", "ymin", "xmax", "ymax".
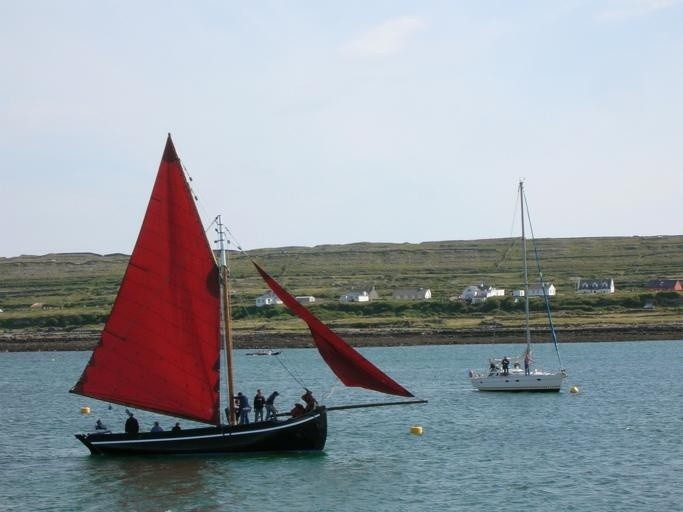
[
  {"xmin": 502, "ymin": 356, "xmax": 509, "ymax": 372},
  {"xmin": 489, "ymin": 359, "xmax": 498, "ymax": 376},
  {"xmin": 266, "ymin": 391, "xmax": 279, "ymax": 421},
  {"xmin": 233, "ymin": 391, "xmax": 251, "ymax": 425},
  {"xmin": 525, "ymin": 354, "xmax": 534, "ymax": 375},
  {"xmin": 292, "ymin": 403, "xmax": 305, "ymax": 417},
  {"xmin": 96, "ymin": 419, "xmax": 107, "ymax": 430},
  {"xmin": 172, "ymin": 422, "xmax": 181, "ymax": 431},
  {"xmin": 254, "ymin": 390, "xmax": 265, "ymax": 423},
  {"xmin": 150, "ymin": 421, "xmax": 163, "ymax": 432},
  {"xmin": 125, "ymin": 413, "xmax": 139, "ymax": 433}
]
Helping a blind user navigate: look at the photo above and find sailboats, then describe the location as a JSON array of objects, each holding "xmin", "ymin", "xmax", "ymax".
[
  {"xmin": 67, "ymin": 131, "xmax": 430, "ymax": 460},
  {"xmin": 468, "ymin": 175, "xmax": 568, "ymax": 392}
]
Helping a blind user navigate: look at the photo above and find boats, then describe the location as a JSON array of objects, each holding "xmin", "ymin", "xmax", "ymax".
[{"xmin": 245, "ymin": 351, "xmax": 281, "ymax": 356}]
